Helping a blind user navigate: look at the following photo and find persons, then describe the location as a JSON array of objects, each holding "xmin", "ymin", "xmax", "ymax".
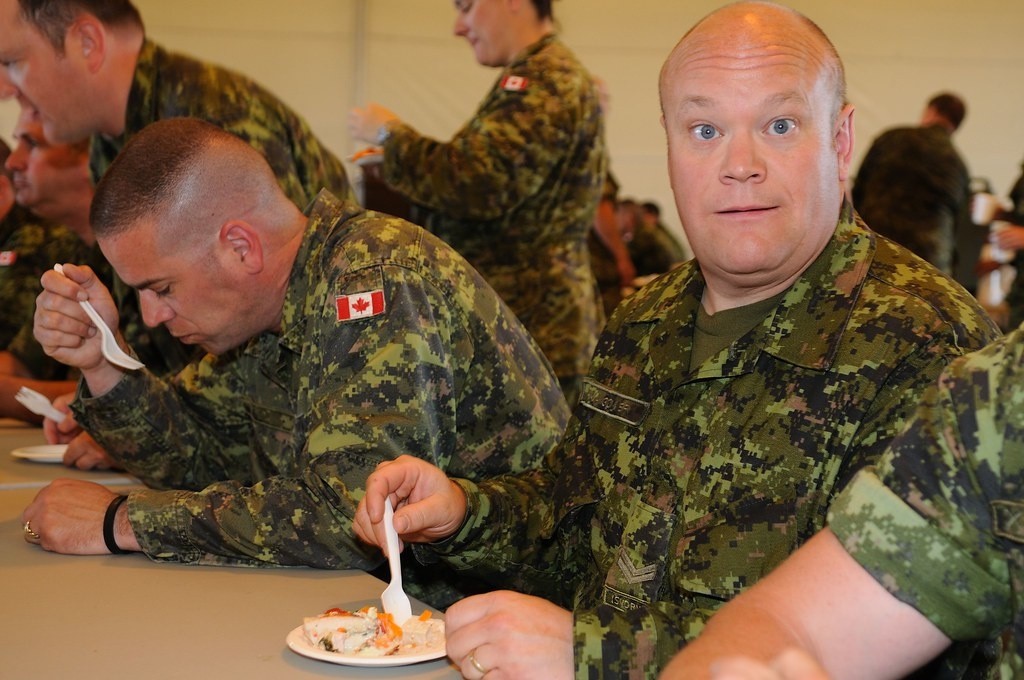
[
  {"xmin": 20, "ymin": 116, "xmax": 572, "ymax": 612},
  {"xmin": 346, "ymin": 0, "xmax": 606, "ymax": 412},
  {"xmin": 991, "ymin": 175, "xmax": 1024, "ymax": 335},
  {"xmin": 352, "ymin": 0, "xmax": 1003, "ymax": 680},
  {"xmin": 0, "ymin": 138, "xmax": 88, "ymax": 350},
  {"xmin": 587, "ymin": 172, "xmax": 685, "ymax": 312},
  {"xmin": 659, "ymin": 323, "xmax": 1023, "ymax": 679},
  {"xmin": 0, "ymin": 116, "xmax": 169, "ymax": 427},
  {"xmin": 850, "ymin": 94, "xmax": 974, "ymax": 277},
  {"xmin": 1, "ymin": 0, "xmax": 359, "ymax": 217}
]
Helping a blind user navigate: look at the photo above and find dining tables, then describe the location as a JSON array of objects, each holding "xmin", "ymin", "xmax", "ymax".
[
  {"xmin": 0, "ymin": 486, "xmax": 468, "ymax": 679},
  {"xmin": 0, "ymin": 413, "xmax": 137, "ymax": 486}
]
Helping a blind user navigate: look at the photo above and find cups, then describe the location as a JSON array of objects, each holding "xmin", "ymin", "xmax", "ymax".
[
  {"xmin": 988, "ymin": 220, "xmax": 1015, "ymax": 263},
  {"xmin": 970, "ymin": 193, "xmax": 997, "ymax": 226}
]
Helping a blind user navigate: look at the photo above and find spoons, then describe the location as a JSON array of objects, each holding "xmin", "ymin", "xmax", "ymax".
[{"xmin": 382, "ymin": 498, "xmax": 412, "ymax": 627}]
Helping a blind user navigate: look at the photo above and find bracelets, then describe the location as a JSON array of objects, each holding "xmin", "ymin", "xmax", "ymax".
[{"xmin": 103, "ymin": 495, "xmax": 128, "ymax": 554}]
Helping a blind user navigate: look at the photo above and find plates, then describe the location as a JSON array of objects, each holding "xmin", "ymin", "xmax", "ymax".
[
  {"xmin": 352, "ymin": 153, "xmax": 385, "ymax": 165},
  {"xmin": 286, "ymin": 614, "xmax": 450, "ymax": 666},
  {"xmin": 10, "ymin": 444, "xmax": 71, "ymax": 462}
]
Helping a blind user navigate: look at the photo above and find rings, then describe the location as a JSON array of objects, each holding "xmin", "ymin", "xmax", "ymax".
[
  {"xmin": 470, "ymin": 649, "xmax": 488, "ymax": 673},
  {"xmin": 24, "ymin": 520, "xmax": 39, "ymax": 539}
]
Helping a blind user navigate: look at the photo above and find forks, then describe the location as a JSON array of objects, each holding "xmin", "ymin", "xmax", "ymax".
[
  {"xmin": 54, "ymin": 263, "xmax": 146, "ymax": 370},
  {"xmin": 14, "ymin": 387, "xmax": 67, "ymax": 422}
]
